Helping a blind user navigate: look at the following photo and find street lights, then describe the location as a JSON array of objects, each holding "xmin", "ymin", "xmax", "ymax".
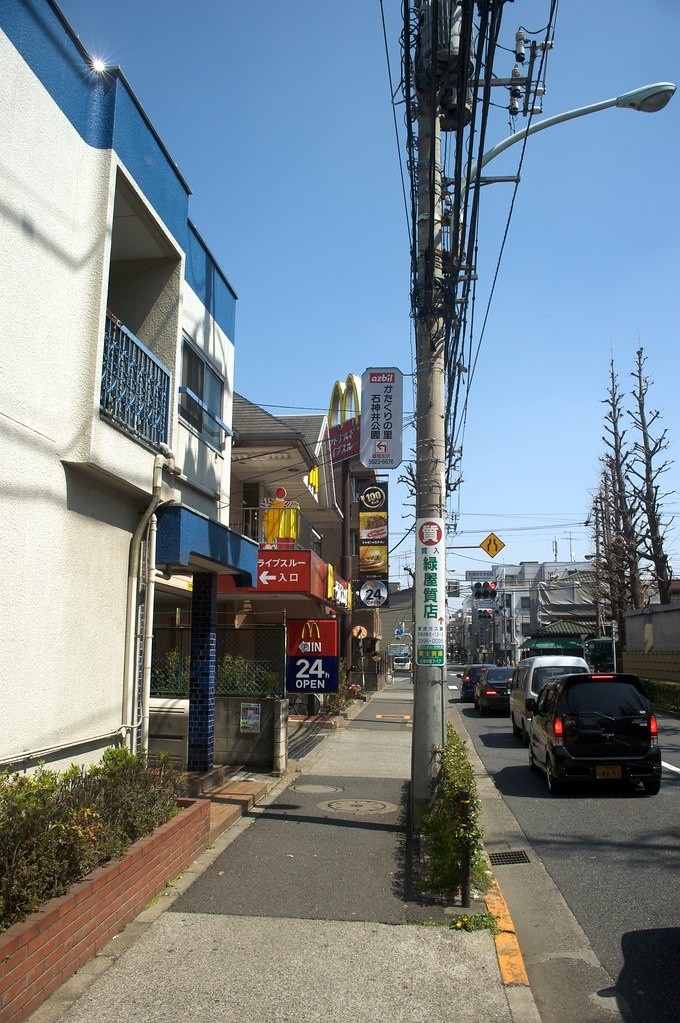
[
  {"xmin": 584, "ymin": 554, "xmax": 617, "ymax": 672},
  {"xmin": 411, "ymin": 82, "xmax": 673, "ymax": 808}
]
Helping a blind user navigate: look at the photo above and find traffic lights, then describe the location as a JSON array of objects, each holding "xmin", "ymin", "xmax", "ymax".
[
  {"xmin": 477, "ymin": 609, "xmax": 493, "ymax": 619},
  {"xmin": 473, "ymin": 581, "xmax": 497, "ymax": 599}
]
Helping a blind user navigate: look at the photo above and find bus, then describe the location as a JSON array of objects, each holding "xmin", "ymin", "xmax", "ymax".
[{"xmin": 584, "ymin": 638, "xmax": 621, "ymax": 673}]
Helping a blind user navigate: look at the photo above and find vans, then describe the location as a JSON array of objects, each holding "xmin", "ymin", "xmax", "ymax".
[
  {"xmin": 393, "ymin": 657, "xmax": 411, "ymax": 672},
  {"xmin": 506, "ymin": 656, "xmax": 592, "ymax": 737}
]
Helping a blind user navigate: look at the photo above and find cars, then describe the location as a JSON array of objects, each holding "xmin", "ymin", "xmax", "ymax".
[
  {"xmin": 457, "ymin": 663, "xmax": 497, "ymax": 701},
  {"xmin": 472, "ymin": 667, "xmax": 516, "ymax": 715},
  {"xmin": 525, "ymin": 672, "xmax": 662, "ymax": 795}
]
